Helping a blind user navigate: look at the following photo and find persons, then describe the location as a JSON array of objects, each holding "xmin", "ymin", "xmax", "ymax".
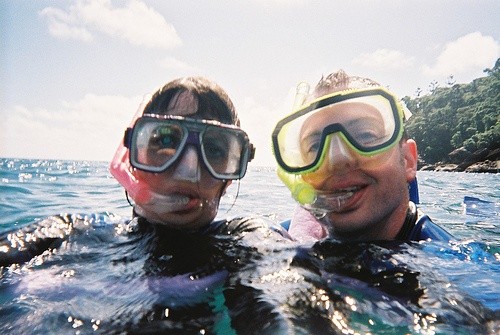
[
  {"xmin": 282, "ymin": 70, "xmax": 499, "ymax": 334},
  {"xmin": 0, "ymin": 76, "xmax": 337, "ymax": 334}
]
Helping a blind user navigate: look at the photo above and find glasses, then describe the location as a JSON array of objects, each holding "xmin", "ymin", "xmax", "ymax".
[
  {"xmin": 123, "ymin": 111, "xmax": 254, "ymax": 181},
  {"xmin": 272, "ymin": 87, "xmax": 405, "ymax": 174}
]
